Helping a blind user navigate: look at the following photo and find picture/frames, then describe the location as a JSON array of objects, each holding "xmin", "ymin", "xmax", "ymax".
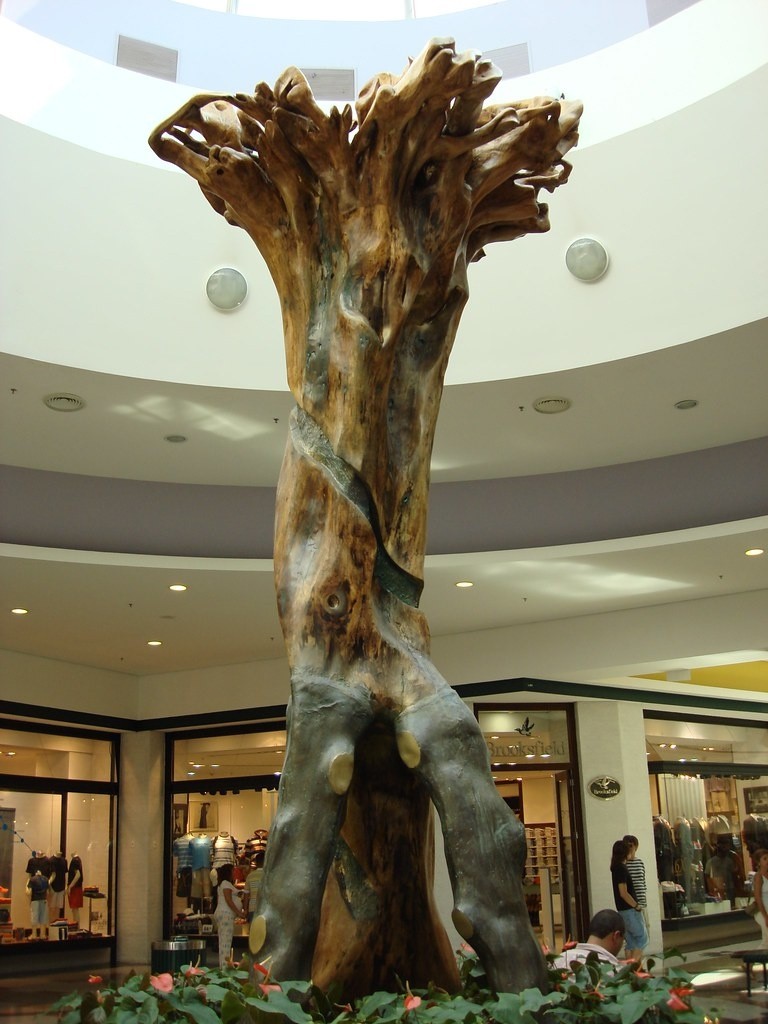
[
  {"xmin": 174, "ymin": 804, "xmax": 188, "ymax": 837},
  {"xmin": 188, "ymin": 801, "xmax": 219, "ymax": 832},
  {"xmin": 743, "ymin": 786, "xmax": 768, "ymax": 814}
]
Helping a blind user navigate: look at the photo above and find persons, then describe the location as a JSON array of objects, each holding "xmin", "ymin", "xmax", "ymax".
[
  {"xmin": 547, "ymin": 908, "xmax": 627, "ymax": 994},
  {"xmin": 173, "ymin": 829, "xmax": 270, "ymax": 912},
  {"xmin": 652, "ymin": 812, "xmax": 768, "ymax": 918},
  {"xmin": 741, "ymin": 848, "xmax": 768, "ymax": 984},
  {"xmin": 213, "ymin": 863, "xmax": 246, "ymax": 977},
  {"xmin": 26, "ymin": 850, "xmax": 84, "ymax": 941},
  {"xmin": 610, "ymin": 835, "xmax": 651, "ymax": 966},
  {"xmin": 704, "ymin": 839, "xmax": 741, "ymax": 908},
  {"xmin": 244, "ymin": 852, "xmax": 265, "ymax": 930}
]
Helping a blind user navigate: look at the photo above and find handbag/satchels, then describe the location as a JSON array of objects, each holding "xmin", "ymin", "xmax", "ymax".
[{"xmin": 746, "ymin": 901, "xmax": 760, "ymax": 916}]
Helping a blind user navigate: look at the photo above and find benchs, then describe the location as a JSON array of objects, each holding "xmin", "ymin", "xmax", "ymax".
[{"xmin": 731, "ymin": 949, "xmax": 768, "ymax": 996}]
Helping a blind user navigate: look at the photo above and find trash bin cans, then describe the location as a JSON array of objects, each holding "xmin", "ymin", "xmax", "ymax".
[
  {"xmin": 170, "ymin": 936, "xmax": 207, "ymax": 968},
  {"xmin": 151, "ymin": 941, "xmax": 187, "ymax": 976}
]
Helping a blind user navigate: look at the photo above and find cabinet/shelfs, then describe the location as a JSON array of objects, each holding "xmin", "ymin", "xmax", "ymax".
[{"xmin": 524, "ymin": 827, "xmax": 558, "ymax": 876}]
